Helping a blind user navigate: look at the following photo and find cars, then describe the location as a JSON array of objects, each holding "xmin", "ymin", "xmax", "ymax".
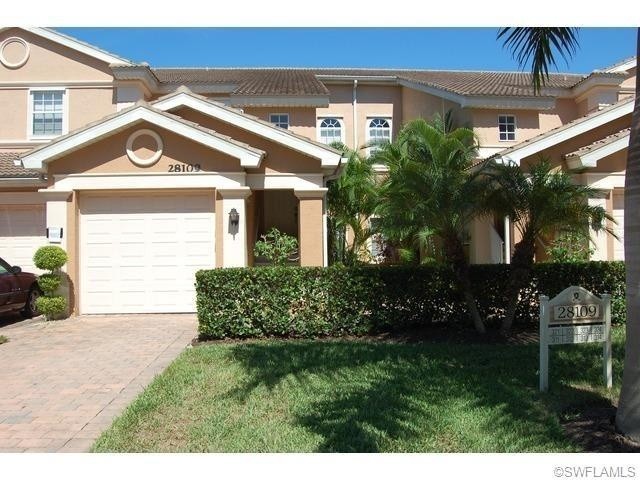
[{"xmin": 0, "ymin": 257, "xmax": 44, "ymax": 323}]
